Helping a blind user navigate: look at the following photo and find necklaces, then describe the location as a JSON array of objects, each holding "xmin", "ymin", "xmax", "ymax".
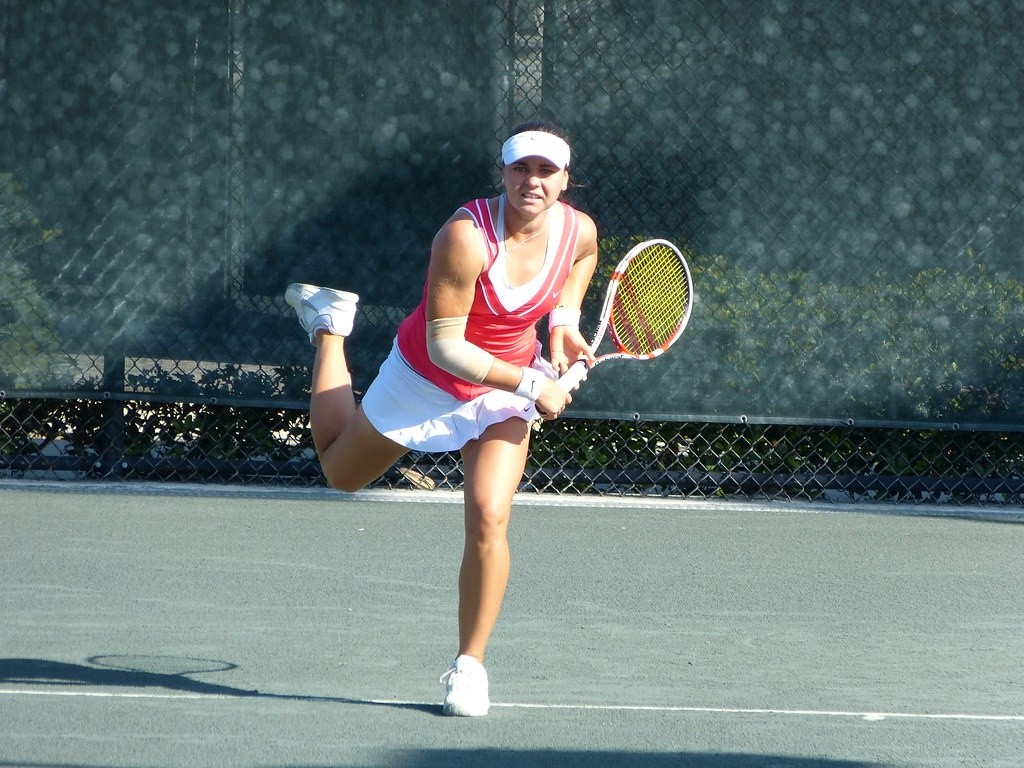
[{"xmin": 504, "ymin": 217, "xmax": 547, "ymax": 263}]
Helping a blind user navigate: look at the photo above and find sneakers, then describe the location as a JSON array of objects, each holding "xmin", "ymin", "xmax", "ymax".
[
  {"xmin": 284, "ymin": 283, "xmax": 360, "ymax": 348},
  {"xmin": 440, "ymin": 655, "xmax": 493, "ymax": 720}
]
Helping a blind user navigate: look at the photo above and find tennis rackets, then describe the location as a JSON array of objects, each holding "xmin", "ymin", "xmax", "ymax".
[{"xmin": 532, "ymin": 238, "xmax": 695, "ymax": 417}]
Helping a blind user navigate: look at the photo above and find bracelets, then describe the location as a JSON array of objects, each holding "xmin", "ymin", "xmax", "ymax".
[
  {"xmin": 548, "ymin": 305, "xmax": 581, "ymax": 333},
  {"xmin": 513, "ymin": 366, "xmax": 545, "ymax": 401}
]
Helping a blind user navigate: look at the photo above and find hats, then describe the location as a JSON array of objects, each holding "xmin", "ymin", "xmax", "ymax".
[{"xmin": 501, "ymin": 130, "xmax": 571, "ymax": 171}]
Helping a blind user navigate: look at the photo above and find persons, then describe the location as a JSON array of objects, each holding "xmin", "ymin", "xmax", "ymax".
[{"xmin": 285, "ymin": 121, "xmax": 599, "ymax": 717}]
